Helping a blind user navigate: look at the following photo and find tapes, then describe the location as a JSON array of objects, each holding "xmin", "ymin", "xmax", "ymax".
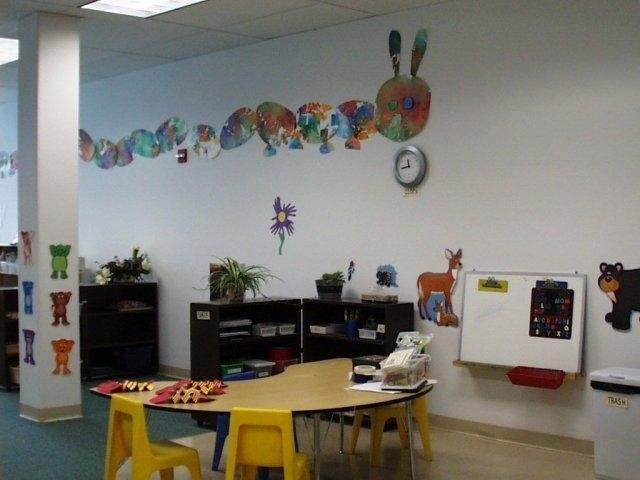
[{"xmin": 353, "ymin": 365, "xmax": 376, "ymax": 374}]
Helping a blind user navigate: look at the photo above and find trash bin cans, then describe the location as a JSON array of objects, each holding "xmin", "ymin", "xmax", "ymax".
[{"xmin": 589, "ymin": 367, "xmax": 640, "ymax": 480}]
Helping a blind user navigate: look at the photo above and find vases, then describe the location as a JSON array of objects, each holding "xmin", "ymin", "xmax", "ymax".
[{"xmin": 112, "ymin": 274, "xmax": 136, "ymax": 283}]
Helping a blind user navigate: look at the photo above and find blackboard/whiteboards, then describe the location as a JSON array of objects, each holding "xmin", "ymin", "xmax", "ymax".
[{"xmin": 458, "ymin": 270, "xmax": 587, "ymax": 376}]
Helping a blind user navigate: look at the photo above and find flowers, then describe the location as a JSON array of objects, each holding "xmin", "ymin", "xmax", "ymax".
[
  {"xmin": 270, "ymin": 196, "xmax": 298, "ymax": 256},
  {"xmin": 91, "ymin": 243, "xmax": 153, "ymax": 285}
]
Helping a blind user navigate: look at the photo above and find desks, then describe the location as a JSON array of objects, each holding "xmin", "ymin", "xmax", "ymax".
[{"xmin": 90, "ymin": 354, "xmax": 438, "ymax": 480}]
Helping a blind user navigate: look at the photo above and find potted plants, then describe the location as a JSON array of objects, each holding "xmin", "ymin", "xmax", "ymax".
[
  {"xmin": 316, "ymin": 271, "xmax": 347, "ymax": 300},
  {"xmin": 192, "ymin": 255, "xmax": 282, "ymax": 305}
]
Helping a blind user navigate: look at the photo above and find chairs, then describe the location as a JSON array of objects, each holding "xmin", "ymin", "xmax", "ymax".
[
  {"xmin": 224, "ymin": 408, "xmax": 312, "ymax": 480},
  {"xmin": 106, "ymin": 391, "xmax": 204, "ymax": 480},
  {"xmin": 349, "ymin": 394, "xmax": 439, "ymax": 468},
  {"xmin": 212, "ymin": 368, "xmax": 300, "ymax": 480}
]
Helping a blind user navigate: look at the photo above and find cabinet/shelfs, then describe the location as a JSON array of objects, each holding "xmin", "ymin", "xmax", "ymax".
[
  {"xmin": 0, "ymin": 258, "xmax": 21, "ymax": 396},
  {"xmin": 188, "ymin": 296, "xmax": 303, "ymax": 431},
  {"xmin": 77, "ymin": 281, "xmax": 160, "ymax": 384},
  {"xmin": 304, "ymin": 295, "xmax": 417, "ymax": 434}
]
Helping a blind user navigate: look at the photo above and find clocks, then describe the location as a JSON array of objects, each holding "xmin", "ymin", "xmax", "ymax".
[{"xmin": 393, "ymin": 146, "xmax": 427, "ymax": 189}]
